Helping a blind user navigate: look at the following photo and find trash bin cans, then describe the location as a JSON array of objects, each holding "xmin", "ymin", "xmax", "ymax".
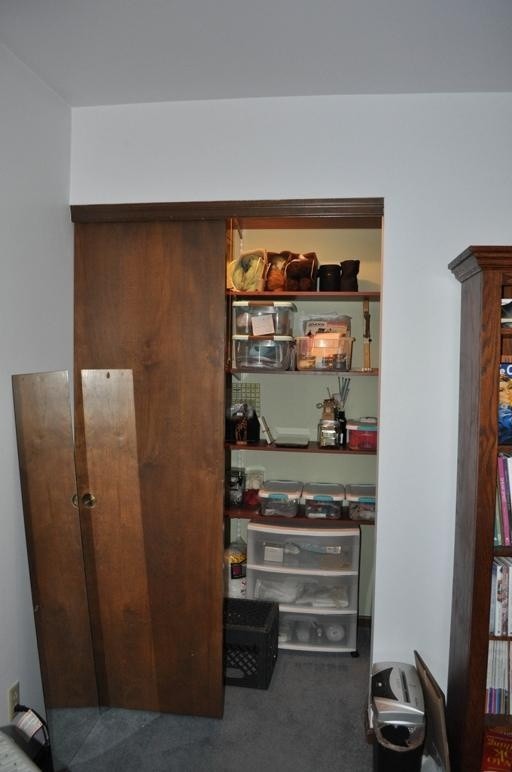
[{"xmin": 373, "ymin": 718, "xmax": 426, "ymax": 771}]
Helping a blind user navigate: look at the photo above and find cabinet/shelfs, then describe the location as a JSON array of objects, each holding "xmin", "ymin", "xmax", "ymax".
[
  {"xmin": 443, "ymin": 243, "xmax": 512, "ymax": 772},
  {"xmin": 63, "ymin": 196, "xmax": 384, "ymax": 717}
]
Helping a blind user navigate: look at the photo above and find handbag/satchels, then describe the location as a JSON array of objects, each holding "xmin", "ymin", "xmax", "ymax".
[{"xmin": 227, "ymin": 248, "xmax": 318, "ymax": 291}]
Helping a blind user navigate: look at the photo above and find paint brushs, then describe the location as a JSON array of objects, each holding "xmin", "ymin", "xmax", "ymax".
[{"xmin": 327, "ymin": 376, "xmax": 351, "ymax": 410}]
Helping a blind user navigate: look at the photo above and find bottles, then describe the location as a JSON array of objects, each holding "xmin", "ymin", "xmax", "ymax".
[{"xmin": 318, "ymin": 399, "xmax": 347, "ymax": 451}]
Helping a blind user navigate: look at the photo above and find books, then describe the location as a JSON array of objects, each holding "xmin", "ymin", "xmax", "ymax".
[{"xmin": 487, "ymin": 298, "xmax": 511, "ymax": 715}]
